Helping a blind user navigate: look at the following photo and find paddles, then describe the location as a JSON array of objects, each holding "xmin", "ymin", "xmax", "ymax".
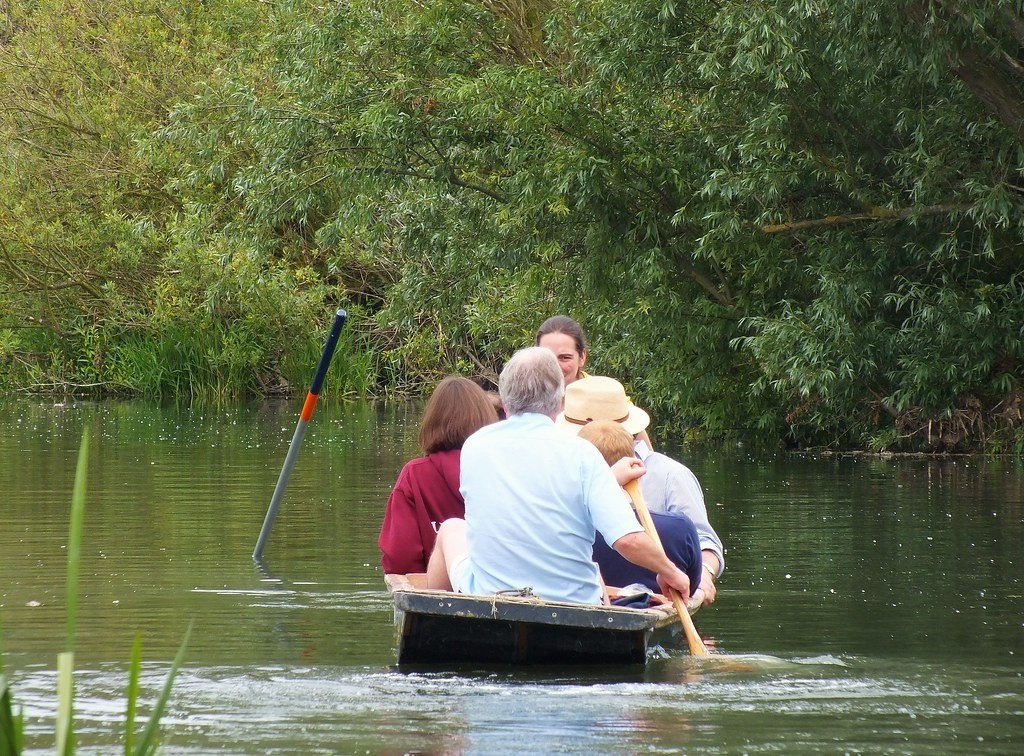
[{"xmin": 622, "ymin": 477, "xmax": 710, "ymax": 656}]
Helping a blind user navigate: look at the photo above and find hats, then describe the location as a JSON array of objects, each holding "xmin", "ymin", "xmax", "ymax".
[{"xmin": 554, "ymin": 376, "xmax": 652, "ymax": 442}]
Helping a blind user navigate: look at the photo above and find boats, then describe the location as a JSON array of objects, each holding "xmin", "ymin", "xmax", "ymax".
[{"xmin": 388, "ymin": 586, "xmax": 710, "ymax": 671}]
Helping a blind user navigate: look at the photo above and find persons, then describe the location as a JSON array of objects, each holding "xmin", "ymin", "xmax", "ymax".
[{"xmin": 379, "ymin": 314, "xmax": 723, "ymax": 606}]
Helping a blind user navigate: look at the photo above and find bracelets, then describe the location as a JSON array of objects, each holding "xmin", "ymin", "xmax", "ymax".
[{"xmin": 702, "ymin": 563, "xmax": 716, "ymax": 583}]
